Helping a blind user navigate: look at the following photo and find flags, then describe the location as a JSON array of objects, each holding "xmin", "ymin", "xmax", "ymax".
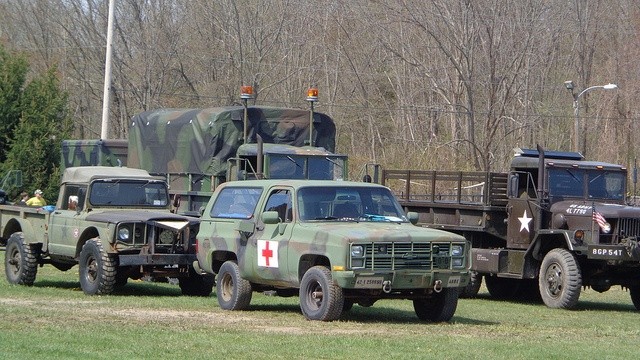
[{"xmin": 593, "ymin": 203, "xmax": 606, "ymax": 230}]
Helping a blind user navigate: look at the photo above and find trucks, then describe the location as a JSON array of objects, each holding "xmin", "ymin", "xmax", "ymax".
[
  {"xmin": 0, "ymin": 166, "xmax": 216, "ymax": 296},
  {"xmin": 364, "ymin": 144, "xmax": 640, "ymax": 308},
  {"xmin": 127, "ymin": 85, "xmax": 347, "ymax": 282}
]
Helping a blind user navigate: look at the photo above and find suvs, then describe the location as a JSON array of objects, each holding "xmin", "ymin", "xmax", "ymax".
[{"xmin": 194, "ymin": 180, "xmax": 470, "ymax": 322}]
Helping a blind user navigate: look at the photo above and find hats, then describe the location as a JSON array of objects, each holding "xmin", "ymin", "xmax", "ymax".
[{"xmin": 35, "ymin": 189, "xmax": 42, "ymax": 193}]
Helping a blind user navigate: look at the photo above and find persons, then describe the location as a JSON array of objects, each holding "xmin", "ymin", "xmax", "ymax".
[{"xmin": 26, "ymin": 189, "xmax": 47, "ymax": 206}]
